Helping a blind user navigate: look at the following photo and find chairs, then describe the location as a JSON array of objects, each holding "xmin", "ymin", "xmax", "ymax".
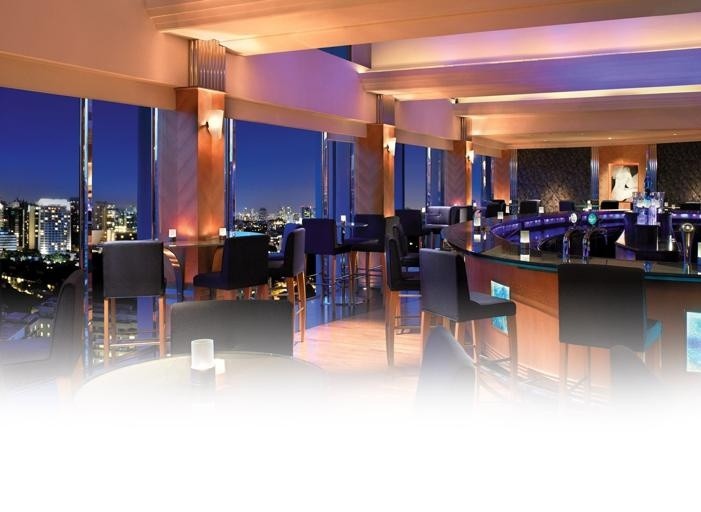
[
  {"xmin": 2, "ymin": 267, "xmax": 89, "ymax": 395},
  {"xmin": 100, "ymin": 198, "xmax": 662, "ymax": 395}
]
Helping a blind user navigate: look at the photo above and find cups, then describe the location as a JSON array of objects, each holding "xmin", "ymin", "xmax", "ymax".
[{"xmin": 190, "ymin": 339, "xmax": 214, "ymax": 371}]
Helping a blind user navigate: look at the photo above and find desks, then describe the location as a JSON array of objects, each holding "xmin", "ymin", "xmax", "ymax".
[{"xmin": 79, "ymin": 351, "xmax": 338, "ymax": 401}]
